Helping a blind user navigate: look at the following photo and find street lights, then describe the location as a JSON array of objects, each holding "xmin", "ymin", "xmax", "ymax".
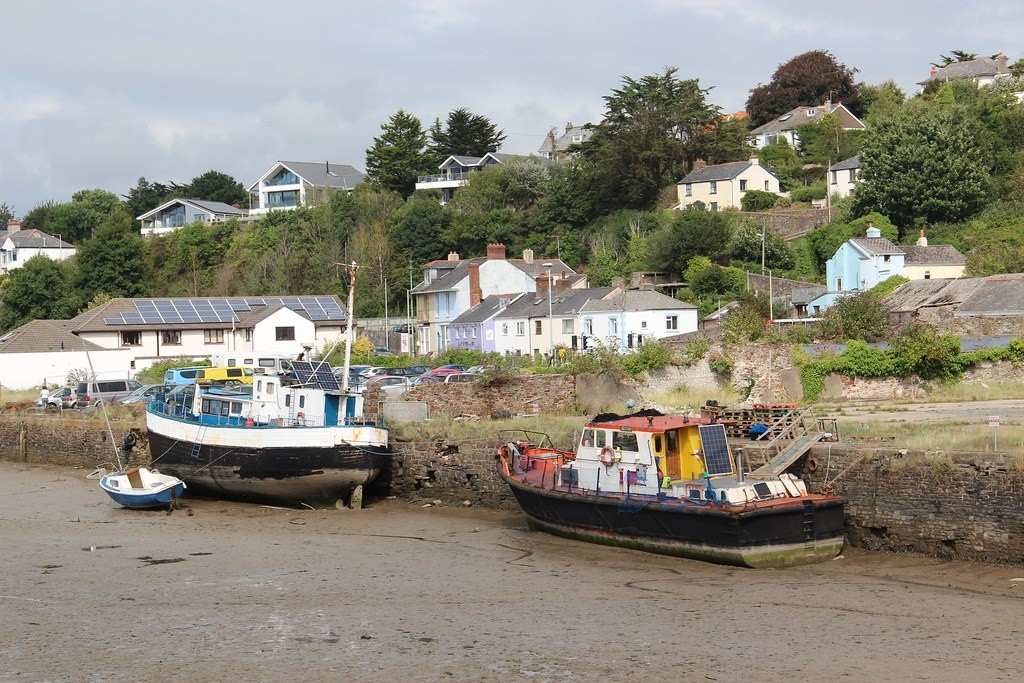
[
  {"xmin": 758, "ymin": 234, "xmax": 765, "ymax": 275},
  {"xmin": 763, "ymin": 269, "xmax": 773, "ymax": 322},
  {"xmin": 543, "ymin": 262, "xmax": 556, "ymax": 367}
]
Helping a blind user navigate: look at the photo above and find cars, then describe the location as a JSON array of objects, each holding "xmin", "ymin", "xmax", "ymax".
[
  {"xmin": 115, "ymin": 384, "xmax": 177, "ymax": 405},
  {"xmin": 166, "ymin": 381, "xmax": 199, "ymax": 409},
  {"xmin": 34, "ymin": 387, "xmax": 64, "ymax": 410},
  {"xmin": 60, "ymin": 387, "xmax": 77, "ymax": 410},
  {"xmin": 332, "ymin": 364, "xmax": 495, "ymax": 394}
]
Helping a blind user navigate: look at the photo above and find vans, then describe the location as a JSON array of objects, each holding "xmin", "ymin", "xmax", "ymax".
[
  {"xmin": 77, "ymin": 380, "xmax": 143, "ymax": 410},
  {"xmin": 196, "ymin": 368, "xmax": 254, "ymax": 387},
  {"xmin": 163, "ymin": 368, "xmax": 199, "ymax": 389}
]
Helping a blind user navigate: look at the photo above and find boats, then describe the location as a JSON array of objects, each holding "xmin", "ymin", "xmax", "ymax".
[
  {"xmin": 77, "ymin": 337, "xmax": 186, "ymax": 508},
  {"xmin": 494, "ymin": 410, "xmax": 847, "ymax": 573},
  {"xmin": 145, "ymin": 263, "xmax": 390, "ymax": 505}
]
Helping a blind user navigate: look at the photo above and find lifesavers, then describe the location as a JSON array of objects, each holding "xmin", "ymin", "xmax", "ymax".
[{"xmin": 599, "ymin": 444, "xmax": 616, "ymax": 468}]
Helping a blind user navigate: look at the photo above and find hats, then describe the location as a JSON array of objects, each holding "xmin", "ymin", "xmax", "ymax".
[{"xmin": 165, "ymin": 397, "xmax": 169, "ymax": 403}]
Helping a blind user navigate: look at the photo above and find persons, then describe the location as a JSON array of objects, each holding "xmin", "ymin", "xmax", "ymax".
[
  {"xmin": 164, "ymin": 388, "xmax": 173, "ymax": 403},
  {"xmin": 122, "ymin": 431, "xmax": 136, "ymax": 464},
  {"xmin": 40, "ymin": 386, "xmax": 49, "ymax": 407}
]
